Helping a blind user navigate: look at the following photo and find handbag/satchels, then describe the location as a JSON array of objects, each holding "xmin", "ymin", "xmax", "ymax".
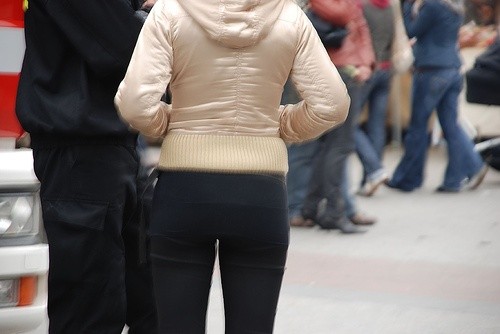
[{"xmin": 307, "ymin": 12, "xmax": 349, "ymax": 49}]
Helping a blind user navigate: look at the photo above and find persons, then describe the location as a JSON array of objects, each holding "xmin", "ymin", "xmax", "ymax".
[
  {"xmin": 115, "ymin": 0, "xmax": 350, "ymax": 334},
  {"xmin": 282, "ymin": 0, "xmax": 488, "ymax": 234},
  {"xmin": 15, "ymin": 0, "xmax": 156, "ymax": 334}
]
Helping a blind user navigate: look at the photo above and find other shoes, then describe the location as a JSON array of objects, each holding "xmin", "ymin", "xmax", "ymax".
[
  {"xmin": 290, "ymin": 216, "xmax": 314, "ymax": 227},
  {"xmin": 351, "ymin": 213, "xmax": 375, "ymax": 225},
  {"xmin": 465, "ymin": 163, "xmax": 489, "ymax": 190},
  {"xmin": 321, "ymin": 217, "xmax": 364, "ymax": 233},
  {"xmin": 357, "ymin": 169, "xmax": 387, "ymax": 195}
]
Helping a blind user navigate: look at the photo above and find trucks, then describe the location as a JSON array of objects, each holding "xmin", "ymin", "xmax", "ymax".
[{"xmin": 0, "ymin": 1, "xmax": 50, "ymax": 333}]
